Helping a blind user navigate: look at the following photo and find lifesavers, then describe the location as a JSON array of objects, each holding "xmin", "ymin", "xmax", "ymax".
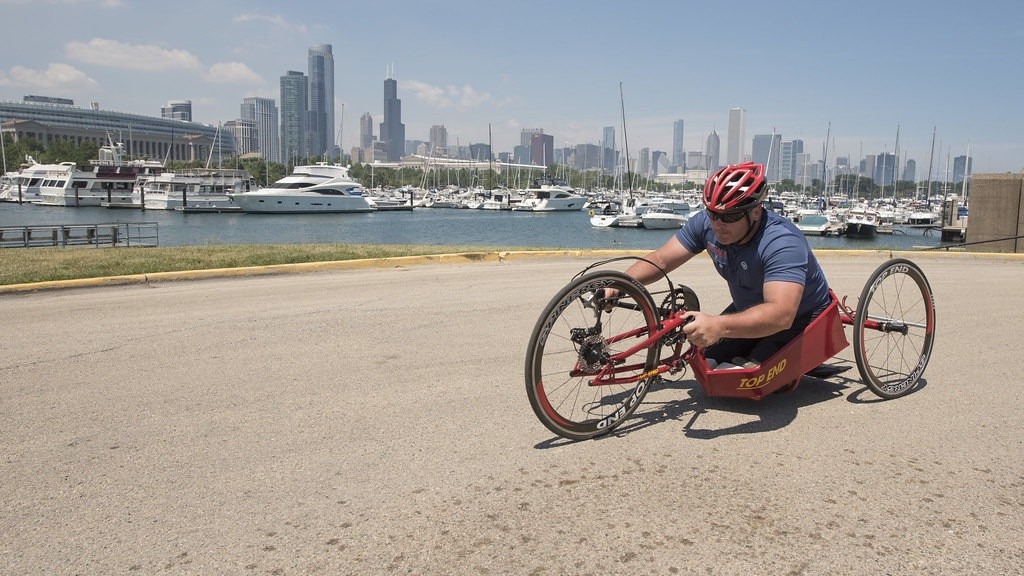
[{"xmin": 588, "ymin": 210, "xmax": 594, "ymax": 216}]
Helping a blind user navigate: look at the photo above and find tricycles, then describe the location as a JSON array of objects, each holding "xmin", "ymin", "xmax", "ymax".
[{"xmin": 522, "ymin": 256, "xmax": 937, "ymax": 444}]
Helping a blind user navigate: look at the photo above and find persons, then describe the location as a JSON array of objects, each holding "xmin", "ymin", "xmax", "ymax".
[{"xmin": 604, "ymin": 162, "xmax": 833, "ymax": 403}]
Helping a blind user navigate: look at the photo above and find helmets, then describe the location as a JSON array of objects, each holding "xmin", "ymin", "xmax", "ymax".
[{"xmin": 702, "ymin": 161, "xmax": 769, "ymax": 213}]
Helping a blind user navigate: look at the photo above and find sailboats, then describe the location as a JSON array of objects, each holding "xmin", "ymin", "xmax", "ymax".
[{"xmin": 0, "ymin": 78, "xmax": 970, "ymax": 238}]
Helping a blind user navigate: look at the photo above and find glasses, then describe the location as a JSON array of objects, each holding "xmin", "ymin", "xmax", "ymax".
[{"xmin": 706, "ymin": 206, "xmax": 756, "ymax": 223}]
